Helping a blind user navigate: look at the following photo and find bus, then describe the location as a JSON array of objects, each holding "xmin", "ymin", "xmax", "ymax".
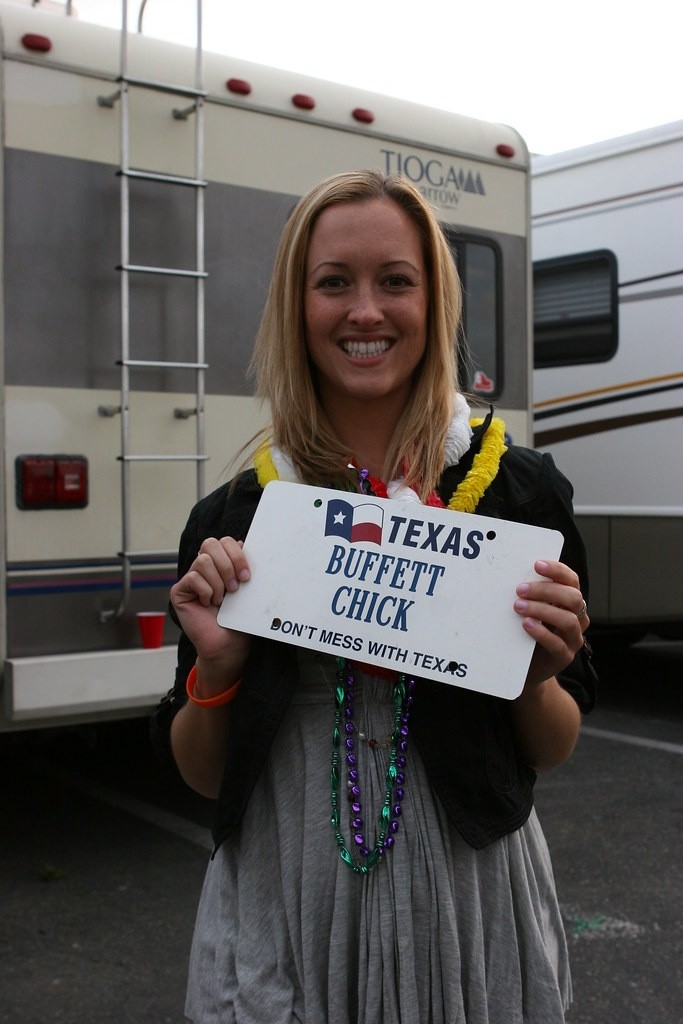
[
  {"xmin": 1, "ymin": 0, "xmax": 532, "ymax": 733},
  {"xmin": 530, "ymin": 117, "xmax": 683, "ymax": 659}
]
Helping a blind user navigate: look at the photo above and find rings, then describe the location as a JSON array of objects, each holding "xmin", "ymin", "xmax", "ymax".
[{"xmin": 576, "ymin": 600, "xmax": 587, "ymax": 617}]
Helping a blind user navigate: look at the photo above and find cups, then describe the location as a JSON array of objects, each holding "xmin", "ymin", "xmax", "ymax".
[{"xmin": 137, "ymin": 612, "xmax": 166, "ymax": 648}]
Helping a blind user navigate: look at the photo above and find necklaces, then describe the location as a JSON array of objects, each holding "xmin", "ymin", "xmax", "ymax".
[{"xmin": 254, "ymin": 393, "xmax": 506, "ymax": 875}]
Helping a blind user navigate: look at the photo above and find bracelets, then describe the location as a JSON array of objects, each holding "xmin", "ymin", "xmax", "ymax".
[{"xmin": 186, "ymin": 667, "xmax": 240, "ymax": 708}]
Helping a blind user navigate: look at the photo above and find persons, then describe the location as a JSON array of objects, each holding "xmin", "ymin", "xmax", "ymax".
[{"xmin": 153, "ymin": 172, "xmax": 596, "ymax": 1024}]
